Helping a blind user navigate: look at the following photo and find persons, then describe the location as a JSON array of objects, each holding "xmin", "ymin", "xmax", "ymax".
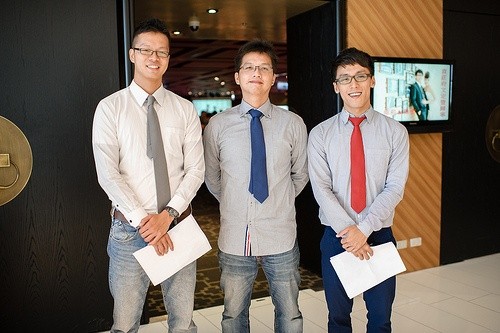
[
  {"xmin": 409, "ymin": 69, "xmax": 429, "ymax": 121},
  {"xmin": 204, "ymin": 41, "xmax": 312, "ymax": 333},
  {"xmin": 423, "ymin": 71, "xmax": 436, "ymax": 121},
  {"xmin": 198, "ymin": 110, "xmax": 222, "ymax": 136},
  {"xmin": 307, "ymin": 47, "xmax": 410, "ymax": 333},
  {"xmin": 91, "ymin": 24, "xmax": 206, "ymax": 333}
]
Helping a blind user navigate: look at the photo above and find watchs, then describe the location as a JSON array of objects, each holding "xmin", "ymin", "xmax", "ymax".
[{"xmin": 163, "ymin": 205, "xmax": 179, "ymax": 218}]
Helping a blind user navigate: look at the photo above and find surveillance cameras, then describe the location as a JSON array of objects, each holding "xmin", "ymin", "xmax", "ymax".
[{"xmin": 188, "ymin": 16, "xmax": 200, "ymax": 31}]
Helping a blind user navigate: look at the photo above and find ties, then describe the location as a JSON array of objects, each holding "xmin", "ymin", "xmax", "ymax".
[
  {"xmin": 248, "ymin": 109, "xmax": 269, "ymax": 203},
  {"xmin": 349, "ymin": 116, "xmax": 367, "ymax": 214},
  {"xmin": 145, "ymin": 96, "xmax": 171, "ymax": 213}
]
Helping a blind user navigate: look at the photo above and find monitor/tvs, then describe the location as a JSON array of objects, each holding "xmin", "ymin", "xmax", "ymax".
[{"xmin": 369, "ymin": 56, "xmax": 456, "ymax": 132}]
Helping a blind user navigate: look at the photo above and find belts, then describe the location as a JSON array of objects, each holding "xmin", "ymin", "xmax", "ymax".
[{"xmin": 114, "ymin": 204, "xmax": 191, "ymax": 228}]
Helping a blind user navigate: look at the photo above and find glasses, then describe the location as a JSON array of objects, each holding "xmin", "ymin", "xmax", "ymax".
[
  {"xmin": 131, "ymin": 47, "xmax": 170, "ymax": 58},
  {"xmin": 335, "ymin": 70, "xmax": 371, "ymax": 84},
  {"xmin": 239, "ymin": 62, "xmax": 273, "ymax": 74}
]
